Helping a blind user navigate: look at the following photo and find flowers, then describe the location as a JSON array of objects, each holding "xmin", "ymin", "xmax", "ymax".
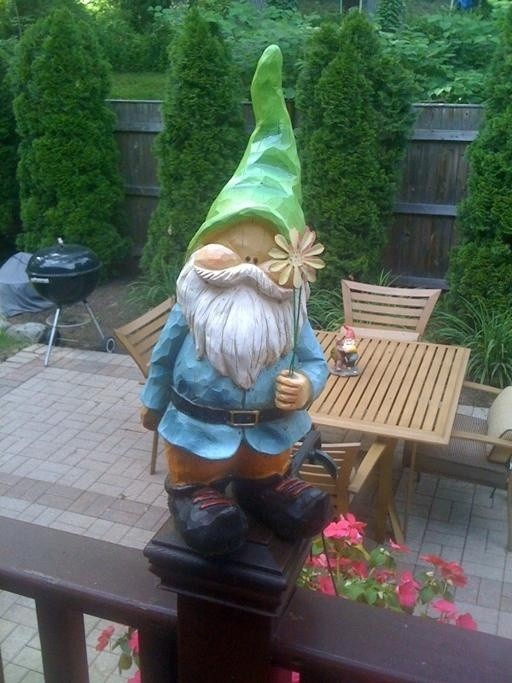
[{"xmin": 93, "ymin": 514, "xmax": 480, "ymax": 683}]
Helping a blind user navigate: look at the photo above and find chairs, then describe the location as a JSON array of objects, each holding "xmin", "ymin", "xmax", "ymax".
[
  {"xmin": 288, "ymin": 434, "xmax": 392, "ymax": 548},
  {"xmin": 391, "ymin": 385, "xmax": 512, "ymax": 554},
  {"xmin": 339, "ymin": 279, "xmax": 443, "ymax": 343},
  {"xmin": 115, "ymin": 294, "xmax": 178, "ymax": 474}
]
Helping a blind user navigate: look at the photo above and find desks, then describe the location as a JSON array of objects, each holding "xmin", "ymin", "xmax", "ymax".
[{"xmin": 302, "ymin": 329, "xmax": 472, "ymax": 551}]
